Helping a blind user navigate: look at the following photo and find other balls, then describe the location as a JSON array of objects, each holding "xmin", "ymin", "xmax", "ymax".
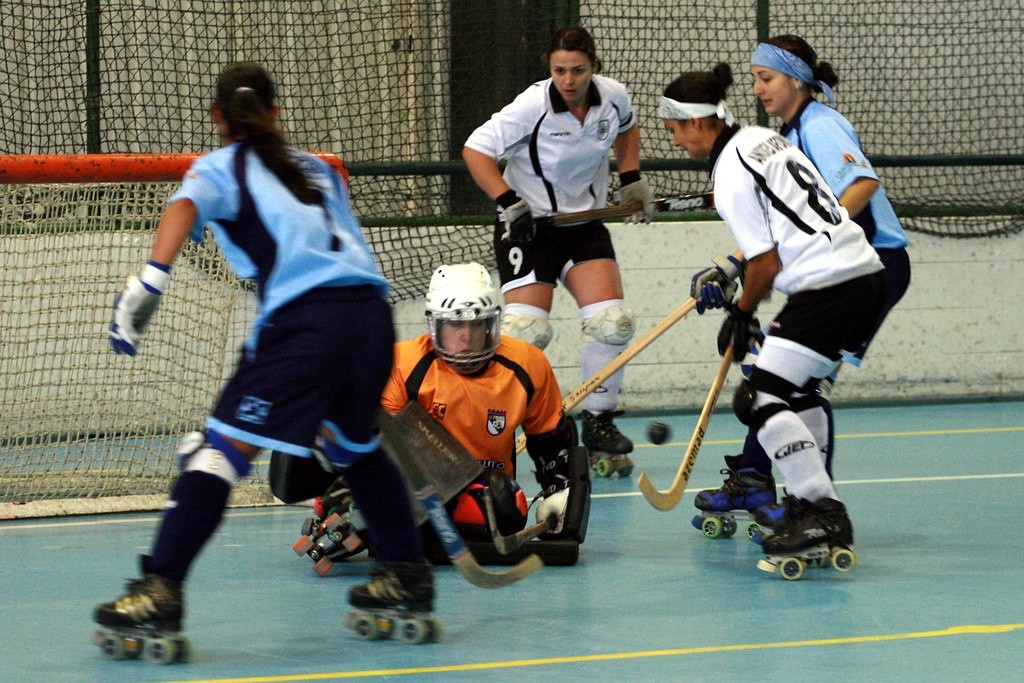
[{"xmin": 650, "ymin": 423, "xmax": 668, "ymax": 445}]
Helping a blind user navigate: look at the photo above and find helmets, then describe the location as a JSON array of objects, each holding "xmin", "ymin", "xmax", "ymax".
[{"xmin": 424, "ymin": 261, "xmax": 499, "ymax": 373}]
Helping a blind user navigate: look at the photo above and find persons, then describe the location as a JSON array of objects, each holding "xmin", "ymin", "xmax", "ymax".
[
  {"xmin": 92, "ymin": 67, "xmax": 435, "ymax": 629},
  {"xmin": 289, "ymin": 263, "xmax": 592, "ymax": 565},
  {"xmin": 660, "ymin": 64, "xmax": 884, "ymax": 553},
  {"xmin": 696, "ymin": 36, "xmax": 912, "ymax": 523},
  {"xmin": 463, "ymin": 28, "xmax": 653, "ymax": 457}
]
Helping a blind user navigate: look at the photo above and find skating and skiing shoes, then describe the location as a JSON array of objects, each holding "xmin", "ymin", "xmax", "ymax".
[
  {"xmin": 346, "ymin": 562, "xmax": 444, "ymax": 645},
  {"xmin": 294, "ymin": 514, "xmax": 363, "ymax": 576},
  {"xmin": 580, "ymin": 409, "xmax": 635, "ymax": 477},
  {"xmin": 757, "ymin": 486, "xmax": 856, "ymax": 580},
  {"xmin": 694, "ymin": 454, "xmax": 785, "ymax": 539},
  {"xmin": 93, "ymin": 573, "xmax": 192, "ymax": 665}
]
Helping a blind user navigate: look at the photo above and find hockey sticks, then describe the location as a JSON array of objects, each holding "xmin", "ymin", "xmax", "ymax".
[
  {"xmin": 533, "ymin": 190, "xmax": 714, "ymax": 231},
  {"xmin": 515, "ymin": 297, "xmax": 698, "ymax": 455},
  {"xmin": 638, "ymin": 334, "xmax": 734, "ymax": 512},
  {"xmin": 378, "ymin": 414, "xmax": 543, "ymax": 589},
  {"xmin": 483, "ymin": 485, "xmax": 556, "ymax": 555}
]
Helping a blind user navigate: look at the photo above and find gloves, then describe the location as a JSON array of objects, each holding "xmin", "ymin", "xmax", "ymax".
[
  {"xmin": 496, "ymin": 189, "xmax": 536, "ymax": 244},
  {"xmin": 718, "ymin": 304, "xmax": 760, "ymax": 363},
  {"xmin": 525, "ymin": 416, "xmax": 592, "ymax": 545},
  {"xmin": 108, "ymin": 260, "xmax": 172, "ymax": 357},
  {"xmin": 690, "ymin": 254, "xmax": 744, "ymax": 315},
  {"xmin": 619, "ymin": 170, "xmax": 656, "ymax": 224}
]
